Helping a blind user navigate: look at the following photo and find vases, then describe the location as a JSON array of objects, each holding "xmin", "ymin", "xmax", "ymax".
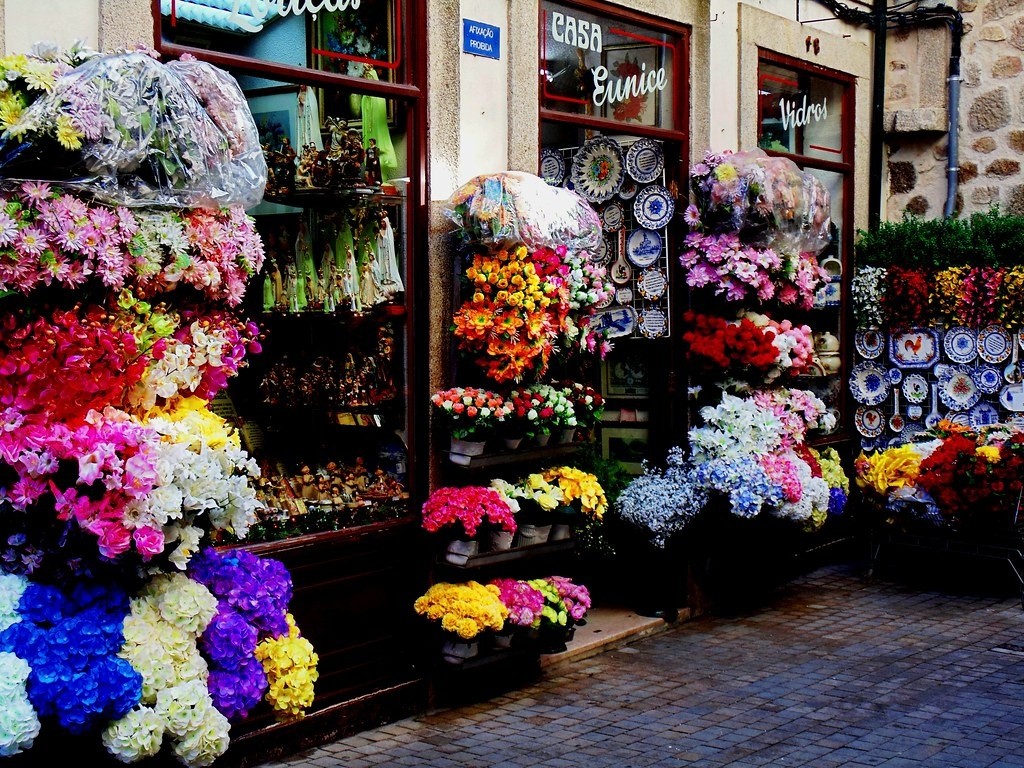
[
  {"xmin": 494, "ymin": 633, "xmax": 513, "ymax": 647},
  {"xmin": 552, "ymin": 628, "xmax": 575, "ymax": 642},
  {"xmin": 451, "ymin": 437, "xmax": 486, "ymax": 456},
  {"xmin": 446, "ymin": 536, "xmax": 478, "ymax": 565},
  {"xmin": 518, "ymin": 525, "xmax": 551, "ymax": 546},
  {"xmin": 581, "ymin": 426, "xmax": 594, "ymax": 440},
  {"xmin": 442, "ymin": 642, "xmax": 478, "ymax": 665},
  {"xmin": 510, "ymin": 628, "xmax": 537, "ymax": 644},
  {"xmin": 554, "ymin": 525, "xmax": 571, "ymax": 540},
  {"xmin": 535, "ymin": 433, "xmax": 551, "ymax": 445},
  {"xmin": 560, "ymin": 428, "xmax": 576, "ymax": 443},
  {"xmin": 492, "ymin": 532, "xmax": 515, "ymax": 552},
  {"xmin": 505, "ymin": 436, "xmax": 522, "ymax": 449}
]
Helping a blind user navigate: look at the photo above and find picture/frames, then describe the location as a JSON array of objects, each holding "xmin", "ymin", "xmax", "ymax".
[
  {"xmin": 590, "ymin": 342, "xmax": 647, "ymax": 412},
  {"xmin": 243, "ymin": 84, "xmax": 306, "ymax": 156},
  {"xmin": 601, "ymin": 39, "xmax": 661, "ymax": 137},
  {"xmin": 758, "ymin": 93, "xmax": 804, "ymax": 171},
  {"xmin": 310, "ymin": 0, "xmax": 401, "ymax": 135}
]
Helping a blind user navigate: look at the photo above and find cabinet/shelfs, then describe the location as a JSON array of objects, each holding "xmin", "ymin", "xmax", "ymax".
[{"xmin": 258, "ymin": 183, "xmax": 405, "ymax": 434}]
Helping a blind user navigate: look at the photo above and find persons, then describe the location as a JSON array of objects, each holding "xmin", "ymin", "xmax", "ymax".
[
  {"xmin": 198, "ymin": 321, "xmax": 421, "ymax": 554},
  {"xmin": 258, "ymin": 114, "xmax": 407, "ymax": 328}
]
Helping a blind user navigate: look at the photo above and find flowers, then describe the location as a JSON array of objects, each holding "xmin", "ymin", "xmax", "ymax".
[
  {"xmin": 542, "ymin": 466, "xmax": 609, "ymax": 526},
  {"xmin": 534, "ymin": 381, "xmax": 578, "ymax": 429},
  {"xmin": 431, "ymin": 387, "xmax": 515, "ymax": 440},
  {"xmin": 515, "ymin": 473, "xmax": 565, "ymax": 525},
  {"xmin": 547, "ymin": 575, "xmax": 591, "ymax": 627},
  {"xmin": 526, "ymin": 579, "xmax": 568, "ymax": 629},
  {"xmin": 422, "ymin": 486, "xmax": 518, "ymax": 536},
  {"xmin": 563, "ymin": 380, "xmax": 606, "ymax": 441},
  {"xmin": 489, "ymin": 479, "xmax": 532, "ymax": 529},
  {"xmin": 414, "ymin": 580, "xmax": 507, "ymax": 640},
  {"xmin": 507, "ymin": 388, "xmax": 553, "ymax": 439},
  {"xmin": 492, "ymin": 578, "xmax": 545, "ymax": 628}
]
